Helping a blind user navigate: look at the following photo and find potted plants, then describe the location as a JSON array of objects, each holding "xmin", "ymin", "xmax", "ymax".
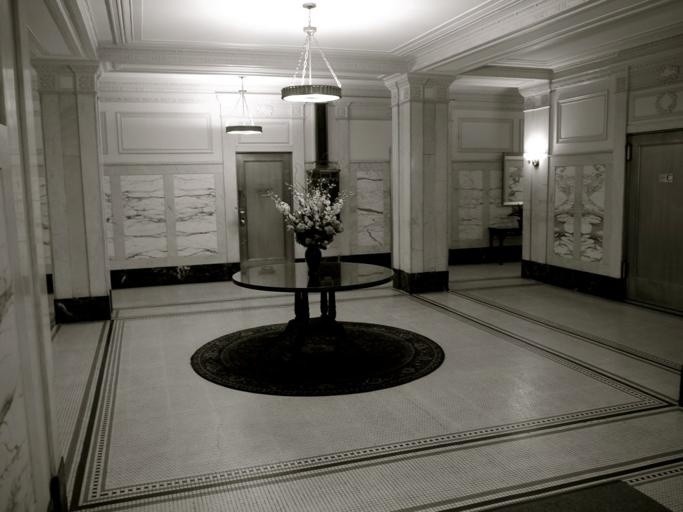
[{"xmin": 506, "ymin": 204, "xmax": 522, "ymax": 228}]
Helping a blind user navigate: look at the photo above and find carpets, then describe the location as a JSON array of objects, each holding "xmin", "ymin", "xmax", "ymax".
[
  {"xmin": 481, "ymin": 478, "xmax": 674, "ymax": 511},
  {"xmin": 189, "ymin": 322, "xmax": 445, "ymax": 396}
]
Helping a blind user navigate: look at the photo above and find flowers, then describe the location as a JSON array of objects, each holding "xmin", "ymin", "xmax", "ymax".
[{"xmin": 265, "ymin": 169, "xmax": 357, "ymax": 250}]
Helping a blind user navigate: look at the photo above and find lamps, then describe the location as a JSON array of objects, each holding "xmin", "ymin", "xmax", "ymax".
[
  {"xmin": 279, "ymin": 3, "xmax": 342, "ymax": 104},
  {"xmin": 225, "ymin": 76, "xmax": 261, "ymax": 136}
]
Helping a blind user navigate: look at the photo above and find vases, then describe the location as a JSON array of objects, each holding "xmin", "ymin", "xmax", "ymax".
[{"xmin": 304, "ymin": 248, "xmax": 321, "ymax": 276}]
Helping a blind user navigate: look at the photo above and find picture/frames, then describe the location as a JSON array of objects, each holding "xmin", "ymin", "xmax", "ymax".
[{"xmin": 501, "ymin": 152, "xmax": 523, "ymax": 207}]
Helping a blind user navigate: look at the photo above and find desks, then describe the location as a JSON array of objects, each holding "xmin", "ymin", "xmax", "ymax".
[
  {"xmin": 487, "ymin": 227, "xmax": 522, "ymax": 265},
  {"xmin": 232, "ymin": 261, "xmax": 397, "ymax": 370}
]
